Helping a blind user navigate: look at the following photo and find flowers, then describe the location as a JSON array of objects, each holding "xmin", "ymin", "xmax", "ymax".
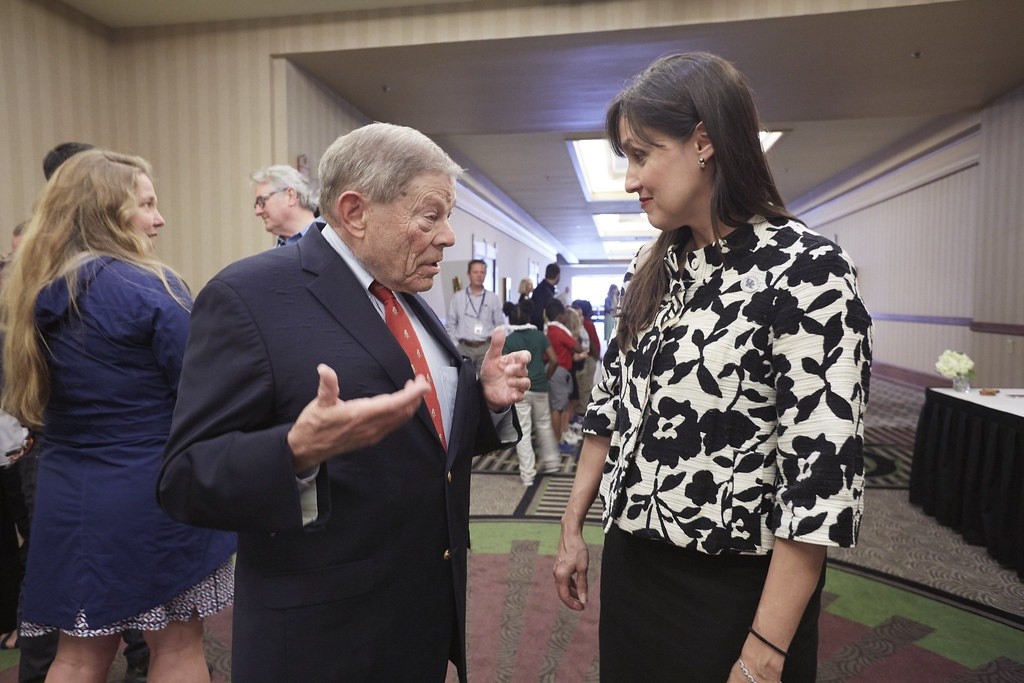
[{"xmin": 935, "ymin": 349, "xmax": 977, "ymax": 381}]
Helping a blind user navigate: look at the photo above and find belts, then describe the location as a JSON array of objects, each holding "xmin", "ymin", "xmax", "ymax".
[{"xmin": 459, "ymin": 337, "xmax": 491, "ymax": 348}]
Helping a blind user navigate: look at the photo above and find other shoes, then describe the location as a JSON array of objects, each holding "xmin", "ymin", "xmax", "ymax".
[{"xmin": 558, "ymin": 415, "xmax": 584, "ymax": 456}]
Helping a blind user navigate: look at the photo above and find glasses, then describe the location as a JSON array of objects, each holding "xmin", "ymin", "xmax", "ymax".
[{"xmin": 254, "ymin": 187, "xmax": 289, "ymax": 208}]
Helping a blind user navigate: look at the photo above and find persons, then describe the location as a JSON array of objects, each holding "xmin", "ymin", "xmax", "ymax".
[
  {"xmin": 552, "ymin": 53, "xmax": 874, "ymax": 683},
  {"xmin": 447, "ymin": 260, "xmax": 621, "ymax": 486},
  {"xmin": 158, "ymin": 122, "xmax": 531, "ymax": 683},
  {"xmin": 0, "ymin": 144, "xmax": 319, "ymax": 683}
]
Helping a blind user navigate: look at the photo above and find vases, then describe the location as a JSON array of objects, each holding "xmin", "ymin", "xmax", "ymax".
[{"xmin": 952, "ymin": 377, "xmax": 970, "ymax": 393}]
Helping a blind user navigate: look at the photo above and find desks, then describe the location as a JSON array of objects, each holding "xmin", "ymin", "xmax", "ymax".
[{"xmin": 908, "ymin": 386, "xmax": 1024, "ymax": 582}]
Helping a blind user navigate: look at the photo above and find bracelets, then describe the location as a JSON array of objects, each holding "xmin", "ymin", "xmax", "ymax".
[
  {"xmin": 749, "ymin": 626, "xmax": 790, "ymax": 658},
  {"xmin": 739, "ymin": 658, "xmax": 782, "ymax": 683}
]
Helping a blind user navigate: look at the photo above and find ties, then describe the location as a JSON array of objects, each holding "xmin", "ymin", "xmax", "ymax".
[{"xmin": 369, "ymin": 279, "xmax": 448, "ymax": 455}]
